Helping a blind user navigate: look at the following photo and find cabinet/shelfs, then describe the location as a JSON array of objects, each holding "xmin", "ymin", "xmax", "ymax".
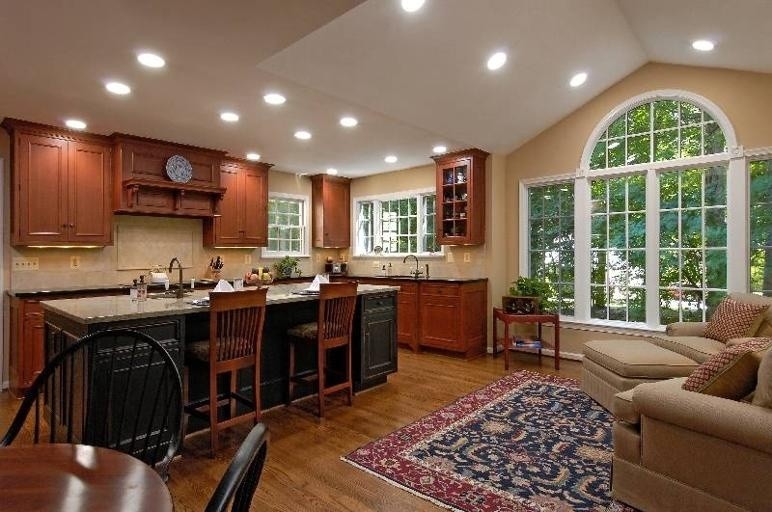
[
  {"xmin": 39, "ymin": 282, "xmax": 402, "ymax": 466},
  {"xmin": 107, "ymin": 131, "xmax": 228, "ymax": 219},
  {"xmin": 1, "ymin": 116, "xmax": 115, "ymax": 247},
  {"xmin": 203, "ymin": 156, "xmax": 274, "ymax": 247},
  {"xmin": 6, "ymin": 276, "xmax": 489, "ymax": 402},
  {"xmin": 431, "ymin": 148, "xmax": 490, "ymax": 247},
  {"xmin": 311, "ymin": 173, "xmax": 351, "ymax": 250}
]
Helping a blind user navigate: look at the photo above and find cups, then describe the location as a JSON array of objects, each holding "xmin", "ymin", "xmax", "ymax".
[
  {"xmin": 322, "ymin": 273, "xmax": 329, "ymax": 284},
  {"xmin": 130, "ymin": 289, "xmax": 138, "ymax": 301},
  {"xmin": 232, "ymin": 274, "xmax": 243, "ymax": 292},
  {"xmin": 137, "ymin": 283, "xmax": 148, "ymax": 302}
]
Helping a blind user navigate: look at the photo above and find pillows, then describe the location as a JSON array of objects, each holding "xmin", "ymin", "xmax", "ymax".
[
  {"xmin": 681, "ymin": 337, "xmax": 772, "ymax": 398},
  {"xmin": 703, "ymin": 293, "xmax": 772, "ymax": 343}
]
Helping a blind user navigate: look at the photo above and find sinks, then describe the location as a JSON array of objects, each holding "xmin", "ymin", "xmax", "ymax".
[{"xmin": 147, "ymin": 292, "xmax": 188, "ymax": 300}]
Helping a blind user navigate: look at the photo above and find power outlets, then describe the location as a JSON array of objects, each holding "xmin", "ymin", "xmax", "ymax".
[
  {"xmin": 70, "ymin": 256, "xmax": 80, "ymax": 269},
  {"xmin": 12, "ymin": 257, "xmax": 39, "ymax": 271}
]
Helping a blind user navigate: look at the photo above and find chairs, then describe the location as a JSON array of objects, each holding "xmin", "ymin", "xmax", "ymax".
[
  {"xmin": 203, "ymin": 422, "xmax": 268, "ymax": 512},
  {"xmin": 0, "ymin": 326, "xmax": 184, "ymax": 483},
  {"xmin": 189, "ymin": 288, "xmax": 268, "ymax": 447},
  {"xmin": 285, "ymin": 280, "xmax": 358, "ymax": 414}
]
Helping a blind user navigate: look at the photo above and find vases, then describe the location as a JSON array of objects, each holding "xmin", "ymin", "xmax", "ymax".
[{"xmin": 502, "ymin": 295, "xmax": 540, "ymax": 314}]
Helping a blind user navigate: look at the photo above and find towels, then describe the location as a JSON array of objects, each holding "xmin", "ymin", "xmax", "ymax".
[
  {"xmin": 309, "ymin": 274, "xmax": 329, "ymax": 292},
  {"xmin": 212, "ymin": 279, "xmax": 236, "ymax": 293}
]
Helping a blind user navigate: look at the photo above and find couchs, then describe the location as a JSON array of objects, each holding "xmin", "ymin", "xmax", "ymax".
[
  {"xmin": 579, "ymin": 291, "xmax": 772, "ymax": 414},
  {"xmin": 612, "ymin": 336, "xmax": 772, "ymax": 512}
]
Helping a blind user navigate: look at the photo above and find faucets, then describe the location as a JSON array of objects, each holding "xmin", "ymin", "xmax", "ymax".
[
  {"xmin": 168, "ymin": 256, "xmax": 184, "ymax": 298},
  {"xmin": 403, "ymin": 255, "xmax": 423, "ymax": 278}
]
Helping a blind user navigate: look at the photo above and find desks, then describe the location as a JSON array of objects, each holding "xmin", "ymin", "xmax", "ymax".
[
  {"xmin": 494, "ymin": 307, "xmax": 561, "ymax": 371},
  {"xmin": 0, "ymin": 443, "xmax": 174, "ymax": 512}
]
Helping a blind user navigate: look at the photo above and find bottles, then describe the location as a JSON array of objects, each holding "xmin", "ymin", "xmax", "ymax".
[
  {"xmin": 130, "ymin": 279, "xmax": 137, "ymax": 301},
  {"xmin": 137, "ymin": 275, "xmax": 147, "ymax": 301},
  {"xmin": 388, "ymin": 263, "xmax": 392, "ymax": 277},
  {"xmin": 382, "ymin": 265, "xmax": 386, "ymax": 277}
]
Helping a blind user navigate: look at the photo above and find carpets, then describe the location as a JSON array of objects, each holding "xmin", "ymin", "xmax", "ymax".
[{"xmin": 341, "ymin": 368, "xmax": 636, "ymax": 512}]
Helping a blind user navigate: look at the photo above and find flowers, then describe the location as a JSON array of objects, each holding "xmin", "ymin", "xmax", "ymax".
[{"xmin": 508, "ymin": 273, "xmax": 575, "ymax": 316}]
